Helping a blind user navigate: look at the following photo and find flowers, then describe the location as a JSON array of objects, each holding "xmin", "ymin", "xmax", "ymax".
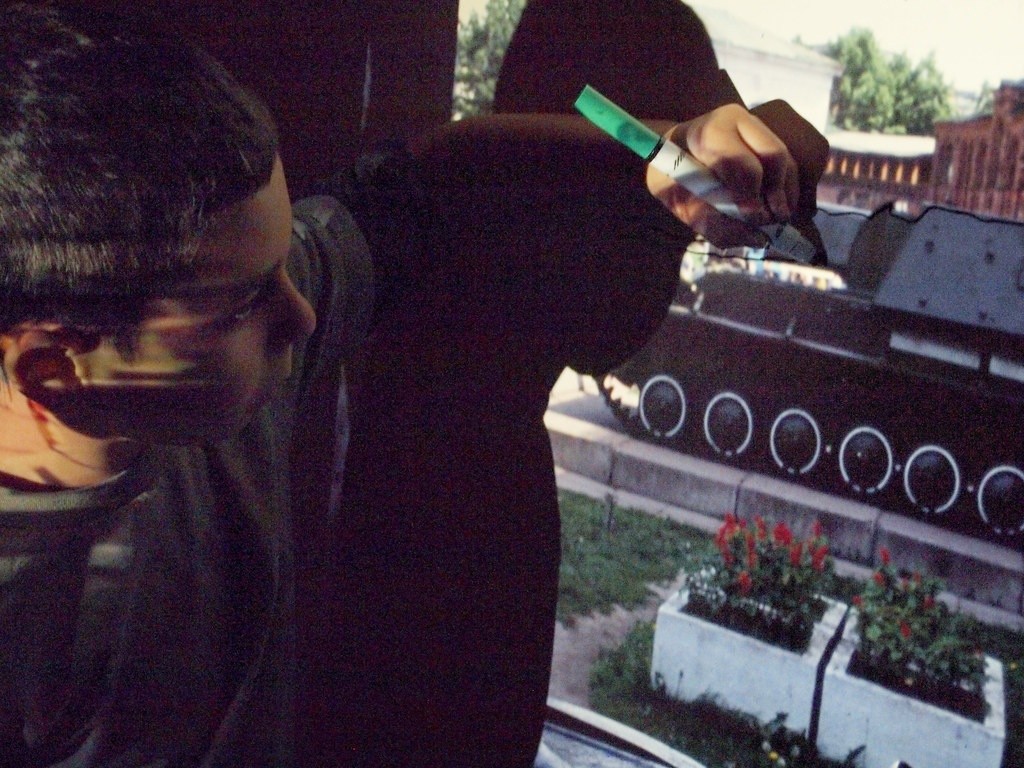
[
  {"xmin": 679, "ymin": 507, "xmax": 837, "ymax": 639},
  {"xmin": 849, "ymin": 542, "xmax": 994, "ymax": 702}
]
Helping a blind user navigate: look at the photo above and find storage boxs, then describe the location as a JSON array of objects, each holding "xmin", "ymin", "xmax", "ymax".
[
  {"xmin": 815, "ymin": 611, "xmax": 1009, "ymax": 768},
  {"xmin": 648, "ymin": 566, "xmax": 855, "ymax": 735}
]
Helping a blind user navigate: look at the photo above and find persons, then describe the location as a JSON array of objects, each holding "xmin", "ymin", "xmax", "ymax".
[{"xmin": 0, "ymin": 0, "xmax": 832, "ymax": 768}]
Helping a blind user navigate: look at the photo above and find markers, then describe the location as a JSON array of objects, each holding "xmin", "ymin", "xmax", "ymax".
[{"xmin": 573, "ymin": 84, "xmax": 827, "ymax": 270}]
